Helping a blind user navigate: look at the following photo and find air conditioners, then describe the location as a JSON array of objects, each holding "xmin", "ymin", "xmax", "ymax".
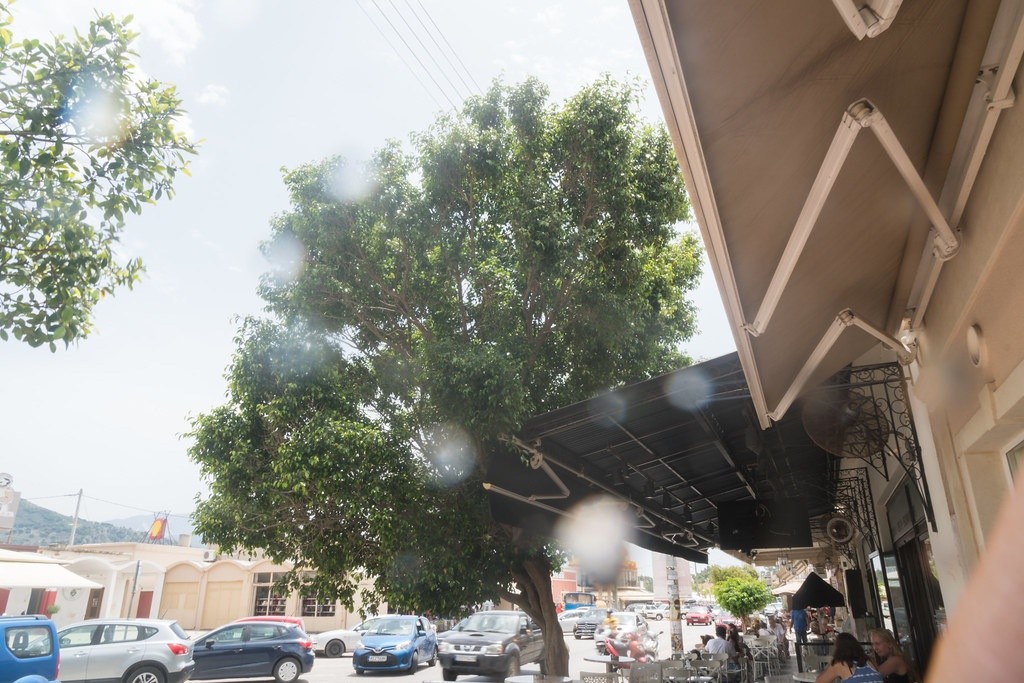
[{"xmin": 202, "ymin": 549, "xmax": 216, "ymax": 561}]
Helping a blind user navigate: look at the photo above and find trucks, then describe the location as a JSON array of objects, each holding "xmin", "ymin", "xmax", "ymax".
[{"xmin": 563, "ymin": 593, "xmax": 597, "ymax": 611}]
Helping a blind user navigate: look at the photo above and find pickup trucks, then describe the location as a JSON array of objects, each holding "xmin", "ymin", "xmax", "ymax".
[
  {"xmin": 647, "ymin": 605, "xmax": 690, "ymax": 621},
  {"xmin": 438, "ymin": 611, "xmax": 548, "ymax": 683}
]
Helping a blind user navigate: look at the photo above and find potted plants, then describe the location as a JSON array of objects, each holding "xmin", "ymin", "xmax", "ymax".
[{"xmin": 47, "ymin": 602, "xmax": 61, "ymax": 614}]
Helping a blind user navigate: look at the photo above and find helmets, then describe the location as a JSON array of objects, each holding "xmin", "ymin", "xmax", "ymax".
[{"xmin": 606, "ymin": 609, "xmax": 613, "ymax": 613}]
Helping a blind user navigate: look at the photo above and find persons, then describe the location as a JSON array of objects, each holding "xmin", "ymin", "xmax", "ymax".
[
  {"xmin": 865, "ymin": 629, "xmax": 911, "ymax": 683},
  {"xmin": 675, "ymin": 604, "xmax": 842, "ymax": 683},
  {"xmin": 815, "ymin": 632, "xmax": 883, "ymax": 683},
  {"xmin": 603, "ymin": 609, "xmax": 618, "ymax": 638}
]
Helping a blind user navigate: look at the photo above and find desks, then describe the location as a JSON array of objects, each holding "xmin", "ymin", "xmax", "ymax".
[
  {"xmin": 792, "ymin": 672, "xmax": 840, "ymax": 683},
  {"xmin": 583, "ymin": 656, "xmax": 636, "ymax": 683},
  {"xmin": 504, "ymin": 674, "xmax": 572, "ymax": 683}
]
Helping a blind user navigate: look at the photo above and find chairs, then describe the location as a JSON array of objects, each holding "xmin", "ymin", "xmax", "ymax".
[
  {"xmin": 622, "ymin": 635, "xmax": 794, "ymax": 683},
  {"xmin": 579, "ymin": 671, "xmax": 619, "ymax": 683},
  {"xmin": 804, "ymin": 654, "xmax": 835, "ymax": 672}
]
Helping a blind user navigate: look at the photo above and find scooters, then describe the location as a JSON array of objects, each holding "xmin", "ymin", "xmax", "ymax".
[
  {"xmin": 602, "ymin": 631, "xmax": 648, "ymax": 671},
  {"xmin": 637, "ymin": 630, "xmax": 664, "ymax": 661}
]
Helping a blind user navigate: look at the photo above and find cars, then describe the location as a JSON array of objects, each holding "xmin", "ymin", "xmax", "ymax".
[
  {"xmin": 745, "ymin": 607, "xmax": 767, "ymax": 624},
  {"xmin": 234, "ymin": 616, "xmax": 306, "ymax": 635},
  {"xmin": 763, "ymin": 605, "xmax": 777, "ymax": 618},
  {"xmin": 685, "ymin": 607, "xmax": 712, "ymax": 626},
  {"xmin": 595, "ymin": 613, "xmax": 650, "ymax": 656},
  {"xmin": 556, "ymin": 610, "xmax": 588, "ymax": 633},
  {"xmin": 575, "ymin": 607, "xmax": 591, "ymax": 615},
  {"xmin": 310, "ymin": 614, "xmax": 401, "ymax": 658},
  {"xmin": 711, "ymin": 604, "xmax": 733, "ymax": 621},
  {"xmin": 22, "ymin": 619, "xmax": 195, "ymax": 683},
  {"xmin": 573, "ymin": 609, "xmax": 613, "ymax": 640},
  {"xmin": 435, "ymin": 616, "xmax": 472, "ymax": 662},
  {"xmin": 188, "ymin": 622, "xmax": 316, "ymax": 683},
  {"xmin": 352, "ymin": 614, "xmax": 439, "ymax": 675},
  {"xmin": 714, "ymin": 612, "xmax": 742, "ymax": 632},
  {"xmin": 633, "ymin": 604, "xmax": 658, "ymax": 616},
  {"xmin": 0, "ymin": 614, "xmax": 63, "ymax": 683}
]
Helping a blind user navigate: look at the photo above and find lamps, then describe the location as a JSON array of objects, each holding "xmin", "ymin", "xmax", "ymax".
[
  {"xmin": 780, "ymin": 548, "xmax": 797, "ymax": 575},
  {"xmin": 612, "ymin": 461, "xmax": 715, "ymax": 537},
  {"xmin": 737, "ymin": 549, "xmax": 758, "ymax": 560}
]
